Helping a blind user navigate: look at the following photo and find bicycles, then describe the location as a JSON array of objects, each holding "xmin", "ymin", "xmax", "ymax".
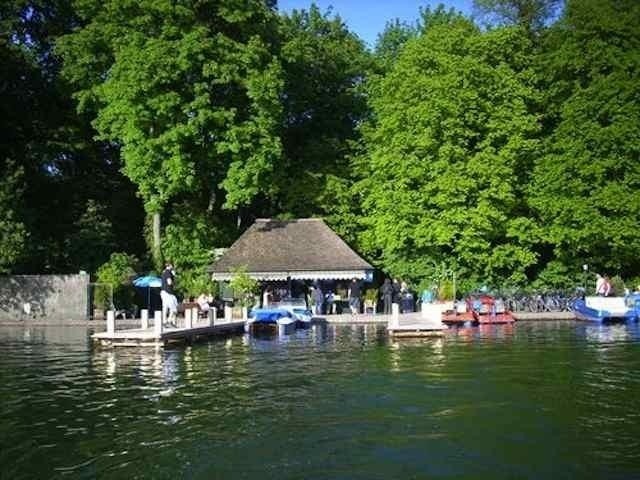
[{"xmin": 503, "ymin": 286, "xmax": 587, "ymax": 314}]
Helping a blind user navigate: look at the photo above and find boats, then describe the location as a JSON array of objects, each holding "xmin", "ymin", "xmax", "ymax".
[
  {"xmin": 243, "ymin": 297, "xmax": 314, "ymax": 337},
  {"xmin": 571, "ymin": 291, "xmax": 640, "ymax": 325}
]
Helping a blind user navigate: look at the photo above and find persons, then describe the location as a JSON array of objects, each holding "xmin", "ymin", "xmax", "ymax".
[
  {"xmin": 309, "ymin": 284, "xmax": 325, "ymax": 316},
  {"xmin": 197, "ymin": 291, "xmax": 210, "ymax": 312},
  {"xmin": 380, "ymin": 275, "xmax": 409, "ymax": 314},
  {"xmin": 159, "ymin": 260, "xmax": 179, "ymax": 328},
  {"xmin": 594, "ymin": 272, "xmax": 610, "ymax": 297},
  {"xmin": 346, "ymin": 278, "xmax": 363, "ymax": 319}
]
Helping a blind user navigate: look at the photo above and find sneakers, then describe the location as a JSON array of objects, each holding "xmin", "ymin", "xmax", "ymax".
[{"xmin": 163, "ymin": 321, "xmax": 179, "ymax": 329}]
